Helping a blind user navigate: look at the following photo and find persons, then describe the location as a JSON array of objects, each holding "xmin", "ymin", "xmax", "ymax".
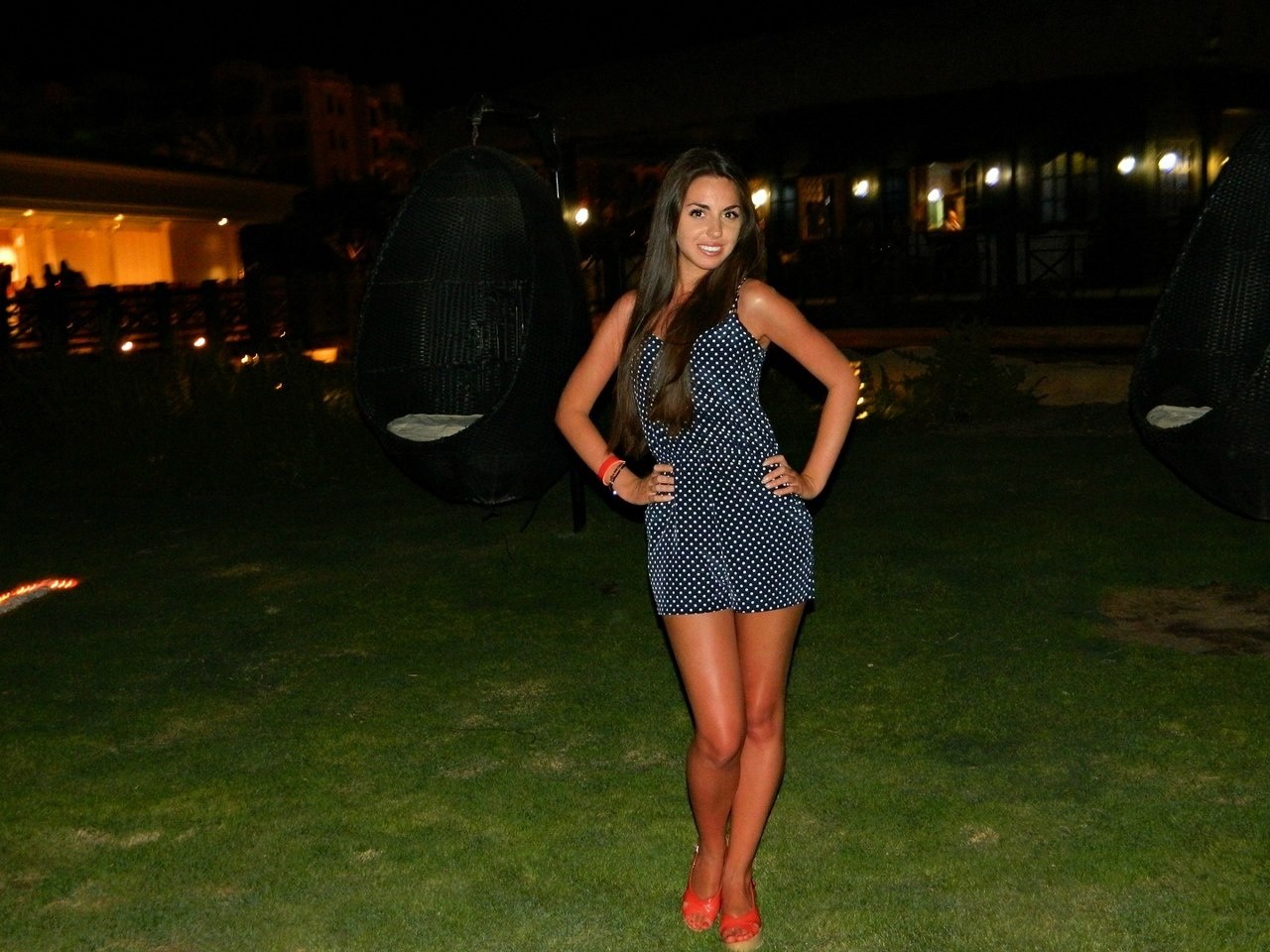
[
  {"xmin": 24, "ymin": 257, "xmax": 87, "ymax": 288},
  {"xmin": 554, "ymin": 145, "xmax": 861, "ymax": 952}
]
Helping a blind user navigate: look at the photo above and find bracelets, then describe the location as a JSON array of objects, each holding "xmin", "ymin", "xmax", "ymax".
[{"xmin": 596, "ymin": 453, "xmax": 626, "ymax": 495}]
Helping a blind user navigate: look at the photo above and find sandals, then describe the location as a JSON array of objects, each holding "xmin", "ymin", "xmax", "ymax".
[
  {"xmin": 682, "ymin": 843, "xmax": 725, "ymax": 933},
  {"xmin": 718, "ymin": 877, "xmax": 763, "ymax": 952}
]
